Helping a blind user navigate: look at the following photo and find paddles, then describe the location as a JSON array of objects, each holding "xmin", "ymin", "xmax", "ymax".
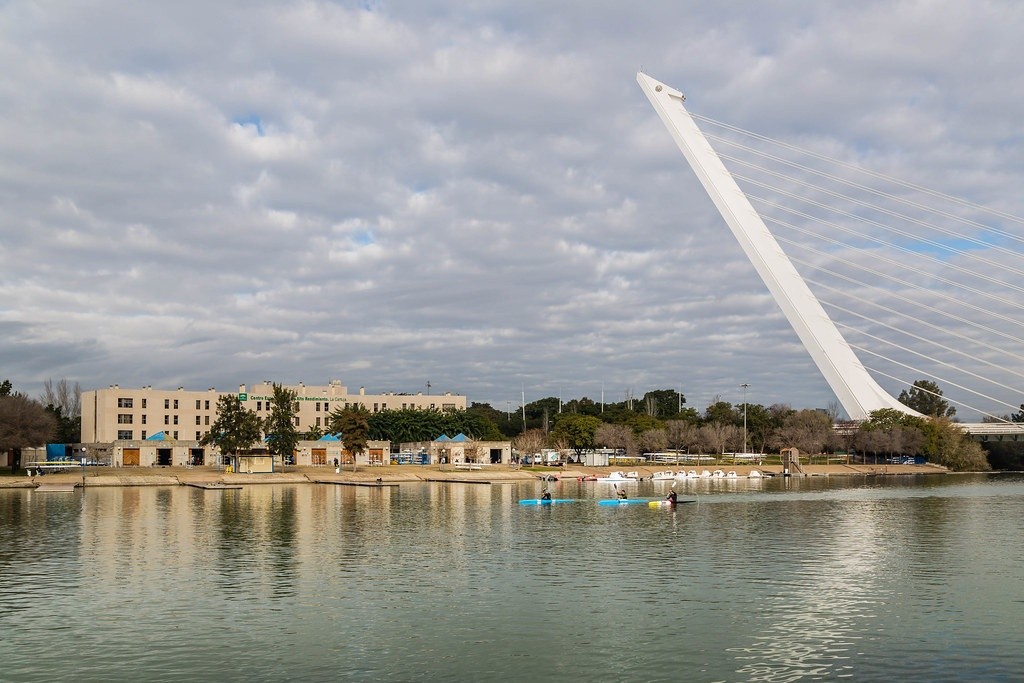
[
  {"xmin": 614, "ymin": 483, "xmax": 620, "ymax": 504},
  {"xmin": 663, "ymin": 482, "xmax": 676, "ymax": 501}
]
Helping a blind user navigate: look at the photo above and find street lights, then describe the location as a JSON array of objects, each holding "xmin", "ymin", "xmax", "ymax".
[{"xmin": 739, "ymin": 383, "xmax": 751, "ymax": 454}]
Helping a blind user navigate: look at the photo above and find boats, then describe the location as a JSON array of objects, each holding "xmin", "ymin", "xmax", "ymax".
[
  {"xmin": 597, "ymin": 498, "xmax": 649, "ymax": 506},
  {"xmin": 599, "ymin": 471, "xmax": 640, "ymax": 482},
  {"xmin": 747, "ymin": 470, "xmax": 761, "ymax": 478},
  {"xmin": 518, "ymin": 498, "xmax": 577, "ymax": 507},
  {"xmin": 577, "ymin": 475, "xmax": 597, "ymax": 482},
  {"xmin": 648, "ymin": 500, "xmax": 696, "ymax": 508},
  {"xmin": 651, "ymin": 469, "xmax": 738, "ymax": 481}
]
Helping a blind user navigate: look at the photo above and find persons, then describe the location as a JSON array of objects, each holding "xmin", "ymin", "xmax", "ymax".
[
  {"xmin": 542, "ymin": 488, "xmax": 551, "ymax": 500},
  {"xmin": 664, "ymin": 488, "xmax": 677, "ymax": 501},
  {"xmin": 617, "ymin": 490, "xmax": 627, "ymax": 500},
  {"xmin": 334, "ymin": 458, "xmax": 339, "ymax": 467}
]
